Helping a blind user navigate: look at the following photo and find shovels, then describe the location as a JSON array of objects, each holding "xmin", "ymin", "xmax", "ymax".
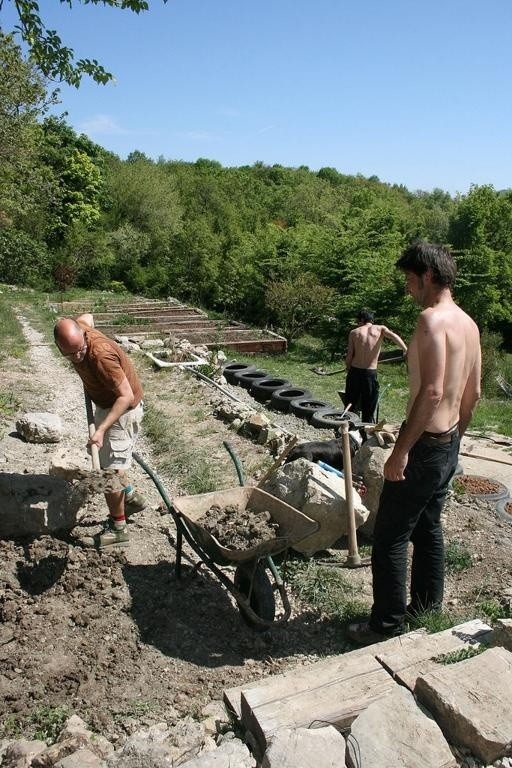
[
  {"xmin": 79, "ymin": 384, "xmax": 129, "ymax": 494},
  {"xmin": 309, "ymin": 357, "xmax": 403, "ymax": 375}
]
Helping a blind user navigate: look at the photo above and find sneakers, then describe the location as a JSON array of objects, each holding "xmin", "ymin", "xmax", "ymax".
[
  {"xmin": 82, "ymin": 524, "xmax": 129, "ymax": 547},
  {"xmin": 346, "ymin": 622, "xmax": 384, "ymax": 643},
  {"xmin": 124, "ymin": 493, "xmax": 148, "ymax": 517}
]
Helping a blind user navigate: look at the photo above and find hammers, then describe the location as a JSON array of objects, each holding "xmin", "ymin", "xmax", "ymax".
[{"xmin": 335, "ymin": 403, "xmax": 353, "ymax": 421}]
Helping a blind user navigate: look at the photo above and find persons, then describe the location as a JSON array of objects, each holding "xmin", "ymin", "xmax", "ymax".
[
  {"xmin": 339, "ymin": 238, "xmax": 481, "ymax": 642},
  {"xmin": 53, "ymin": 311, "xmax": 149, "ymax": 547},
  {"xmin": 344, "ymin": 306, "xmax": 408, "ymax": 428}
]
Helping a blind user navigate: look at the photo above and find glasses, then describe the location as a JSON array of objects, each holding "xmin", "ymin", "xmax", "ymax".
[{"xmin": 56, "ymin": 341, "xmax": 87, "ymax": 356}]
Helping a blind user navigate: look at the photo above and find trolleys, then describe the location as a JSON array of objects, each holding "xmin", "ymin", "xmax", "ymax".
[{"xmin": 131, "ymin": 439, "xmax": 321, "ymax": 634}]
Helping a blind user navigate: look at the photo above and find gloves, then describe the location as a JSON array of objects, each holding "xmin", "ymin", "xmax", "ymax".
[{"xmin": 364, "ymin": 418, "xmax": 399, "ymax": 446}]
